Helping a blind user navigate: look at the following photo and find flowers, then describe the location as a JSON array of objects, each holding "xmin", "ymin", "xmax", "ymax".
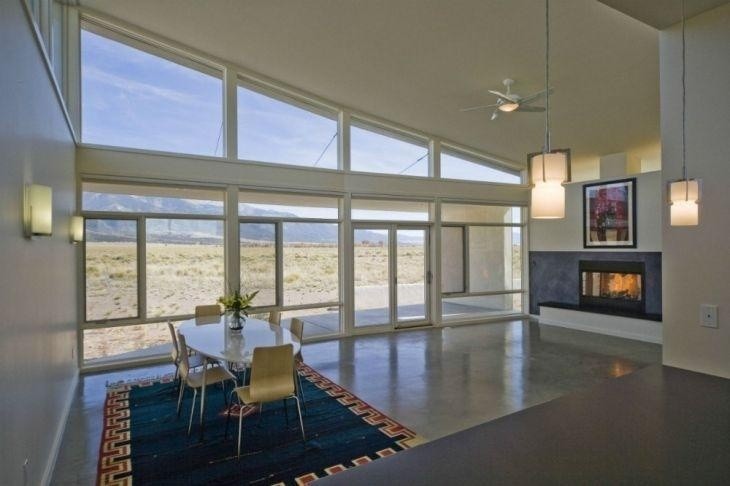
[{"xmin": 216, "ymin": 288, "xmax": 260, "ymax": 322}]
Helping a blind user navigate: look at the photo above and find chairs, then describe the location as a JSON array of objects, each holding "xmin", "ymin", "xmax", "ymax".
[{"xmin": 167, "ymin": 305, "xmax": 306, "ymax": 459}]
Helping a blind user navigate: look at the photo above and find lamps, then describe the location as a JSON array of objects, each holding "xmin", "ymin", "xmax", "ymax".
[
  {"xmin": 25, "ymin": 183, "xmax": 53, "ymax": 238},
  {"xmin": 669, "ymin": 0, "xmax": 701, "ymax": 228},
  {"xmin": 69, "ymin": 214, "xmax": 86, "ymax": 245},
  {"xmin": 530, "ymin": 0, "xmax": 567, "ymax": 221}
]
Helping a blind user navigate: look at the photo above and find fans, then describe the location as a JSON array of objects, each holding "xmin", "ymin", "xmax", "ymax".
[{"xmin": 457, "ymin": 78, "xmax": 557, "ymax": 122}]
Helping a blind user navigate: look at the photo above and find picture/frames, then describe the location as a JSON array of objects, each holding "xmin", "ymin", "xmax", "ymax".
[{"xmin": 581, "ymin": 175, "xmax": 638, "ymax": 250}]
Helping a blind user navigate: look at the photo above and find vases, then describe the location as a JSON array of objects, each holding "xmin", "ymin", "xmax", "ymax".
[{"xmin": 227, "ymin": 308, "xmax": 247, "ymax": 331}]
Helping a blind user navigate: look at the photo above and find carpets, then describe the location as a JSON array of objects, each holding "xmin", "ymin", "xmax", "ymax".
[{"xmin": 94, "ymin": 357, "xmax": 430, "ymax": 485}]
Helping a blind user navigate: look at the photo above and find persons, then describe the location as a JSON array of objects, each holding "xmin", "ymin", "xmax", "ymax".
[
  {"xmin": 595, "ymin": 188, "xmax": 611, "ymax": 241},
  {"xmin": 615, "ymin": 190, "xmax": 627, "ymax": 240}
]
